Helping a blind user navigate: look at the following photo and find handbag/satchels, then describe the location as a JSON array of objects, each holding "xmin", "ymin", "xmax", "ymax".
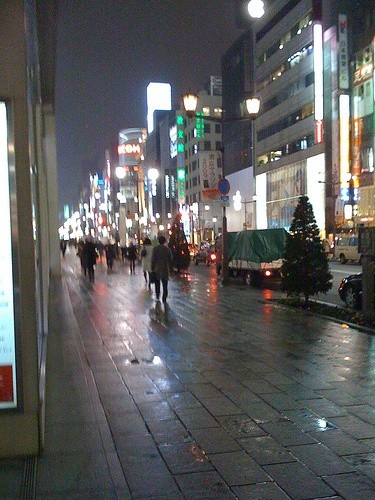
[
  {"xmin": 141, "ymin": 245, "xmax": 147, "ymax": 256},
  {"xmin": 150, "ymin": 272, "xmax": 157, "ymax": 283}
]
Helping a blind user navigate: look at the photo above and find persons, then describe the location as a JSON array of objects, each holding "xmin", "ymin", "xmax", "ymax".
[{"xmin": 58, "ymin": 236, "xmax": 174, "ymax": 302}]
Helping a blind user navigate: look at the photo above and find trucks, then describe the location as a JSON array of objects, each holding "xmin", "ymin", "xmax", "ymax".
[{"xmin": 215, "ymin": 227, "xmax": 290, "ymax": 285}]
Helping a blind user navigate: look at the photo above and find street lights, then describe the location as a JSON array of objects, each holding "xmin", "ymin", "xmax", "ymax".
[
  {"xmin": 316, "ymin": 171, "xmax": 352, "ymax": 246},
  {"xmin": 232, "ymin": 194, "xmax": 257, "ymax": 230},
  {"xmin": 180, "ymin": 89, "xmax": 261, "ymax": 286},
  {"xmin": 116, "ymin": 167, "xmax": 159, "ymax": 253}
]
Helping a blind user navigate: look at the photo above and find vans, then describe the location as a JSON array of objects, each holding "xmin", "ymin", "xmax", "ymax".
[{"xmin": 334, "ymin": 234, "xmax": 363, "ymax": 265}]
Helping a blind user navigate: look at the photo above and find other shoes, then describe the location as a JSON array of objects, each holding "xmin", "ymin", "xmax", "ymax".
[
  {"xmin": 156, "ymin": 296, "xmax": 159, "ymax": 300},
  {"xmin": 162, "ymin": 296, "xmax": 167, "ymax": 302}
]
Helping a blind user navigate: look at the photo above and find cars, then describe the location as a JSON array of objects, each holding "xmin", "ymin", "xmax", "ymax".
[
  {"xmin": 337, "ymin": 272, "xmax": 363, "ymax": 309},
  {"xmin": 187, "ymin": 244, "xmax": 199, "ymax": 256},
  {"xmin": 192, "ymin": 252, "xmax": 219, "ymax": 265}
]
